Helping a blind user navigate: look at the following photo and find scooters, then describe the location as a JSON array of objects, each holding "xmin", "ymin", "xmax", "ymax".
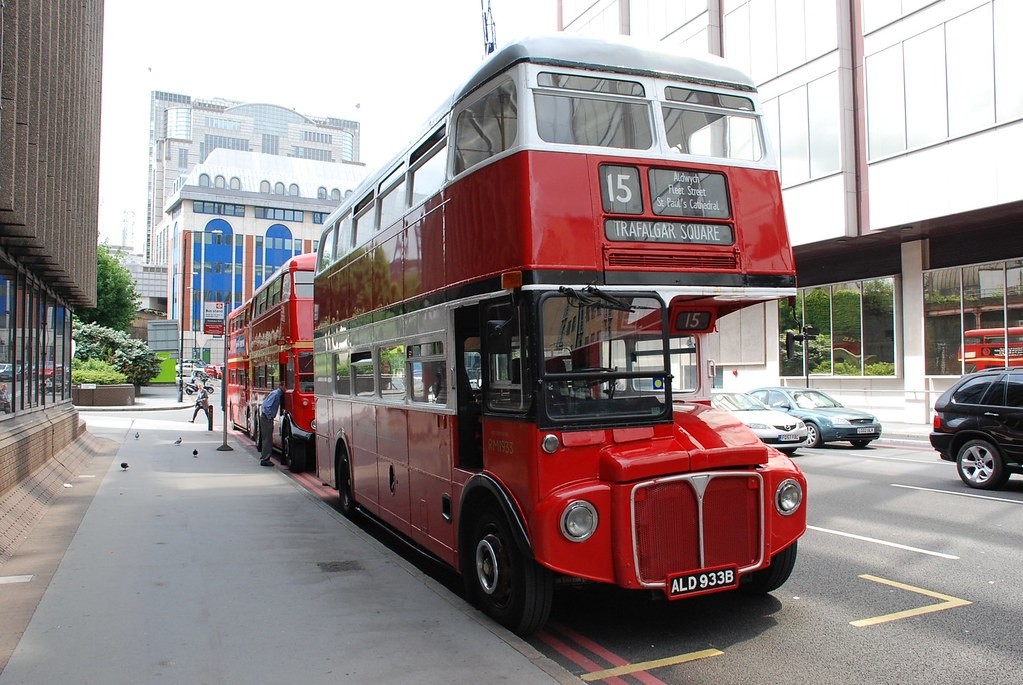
[
  {"xmin": 184, "ymin": 374, "xmax": 215, "ymax": 395},
  {"xmin": 39, "ymin": 374, "xmax": 69, "ymax": 394}
]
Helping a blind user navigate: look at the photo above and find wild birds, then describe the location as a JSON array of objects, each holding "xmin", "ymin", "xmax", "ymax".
[
  {"xmin": 135, "ymin": 432, "xmax": 139, "ymax": 438},
  {"xmin": 174, "ymin": 436, "xmax": 182, "ymax": 445},
  {"xmin": 121, "ymin": 463, "xmax": 129, "ymax": 470},
  {"xmin": 193, "ymin": 449, "xmax": 198, "ymax": 456}
]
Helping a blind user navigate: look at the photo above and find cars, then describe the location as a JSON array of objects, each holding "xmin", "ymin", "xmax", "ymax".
[
  {"xmin": 176, "ymin": 362, "xmax": 204, "ymax": 377},
  {"xmin": 205, "ymin": 364, "xmax": 224, "ymax": 379},
  {"xmin": 0, "ymin": 365, "xmax": 27, "ymax": 381},
  {"xmin": 680, "ymin": 388, "xmax": 809, "ymax": 453},
  {"xmin": 749, "ymin": 387, "xmax": 882, "ymax": 448},
  {"xmin": 37, "ymin": 361, "xmax": 63, "ymax": 376}
]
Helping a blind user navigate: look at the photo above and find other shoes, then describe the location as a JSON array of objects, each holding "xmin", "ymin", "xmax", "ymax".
[
  {"xmin": 188, "ymin": 420, "xmax": 194, "ymax": 422},
  {"xmin": 260, "ymin": 460, "xmax": 275, "ymax": 466}
]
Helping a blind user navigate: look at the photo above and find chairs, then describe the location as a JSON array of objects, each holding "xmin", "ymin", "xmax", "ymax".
[{"xmin": 509, "ymin": 355, "xmax": 568, "ymax": 406}]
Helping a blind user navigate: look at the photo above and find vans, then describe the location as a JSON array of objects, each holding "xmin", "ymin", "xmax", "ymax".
[{"xmin": 182, "ymin": 358, "xmax": 206, "ymax": 366}]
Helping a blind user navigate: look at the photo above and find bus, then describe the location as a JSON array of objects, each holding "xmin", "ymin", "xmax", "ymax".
[
  {"xmin": 310, "ymin": 36, "xmax": 809, "ymax": 640},
  {"xmin": 959, "ymin": 326, "xmax": 1023, "ymax": 371},
  {"xmin": 224, "ymin": 253, "xmax": 318, "ymax": 473}
]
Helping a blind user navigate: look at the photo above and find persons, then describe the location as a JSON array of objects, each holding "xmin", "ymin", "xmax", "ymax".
[
  {"xmin": 190, "ymin": 363, "xmax": 194, "ymax": 377},
  {"xmin": 176, "ymin": 362, "xmax": 180, "ymax": 377},
  {"xmin": 414, "ymin": 314, "xmax": 473, "ymax": 467},
  {"xmin": 188, "ymin": 384, "xmax": 209, "ymax": 423},
  {"xmin": 0, "ymin": 384, "xmax": 11, "ymax": 414},
  {"xmin": 213, "ymin": 366, "xmax": 219, "ymax": 381},
  {"xmin": 259, "ymin": 380, "xmax": 285, "ymax": 467}
]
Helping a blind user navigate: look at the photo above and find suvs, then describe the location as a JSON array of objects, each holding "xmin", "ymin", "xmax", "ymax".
[{"xmin": 928, "ymin": 365, "xmax": 1023, "ymax": 489}]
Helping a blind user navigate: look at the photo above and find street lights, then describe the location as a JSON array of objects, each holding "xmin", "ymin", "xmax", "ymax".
[
  {"xmin": 171, "ymin": 272, "xmax": 198, "ymax": 320},
  {"xmin": 178, "ymin": 230, "xmax": 224, "ymax": 402}
]
